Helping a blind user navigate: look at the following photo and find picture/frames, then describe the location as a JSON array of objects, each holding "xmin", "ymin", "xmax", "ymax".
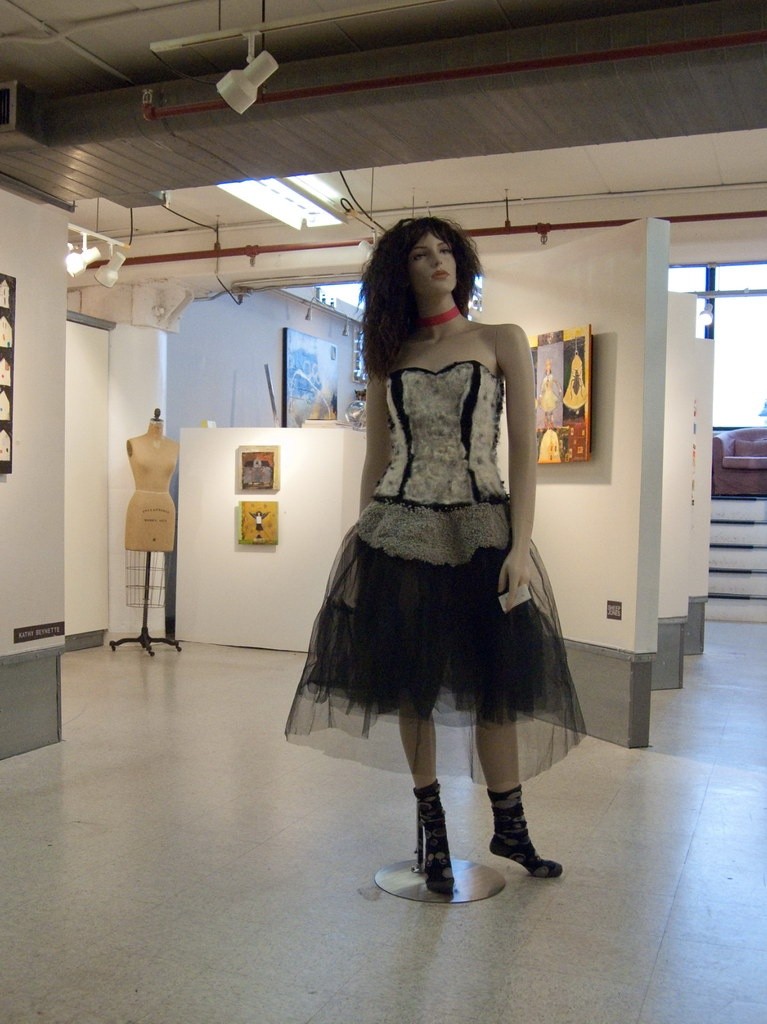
[
  {"xmin": 238, "ymin": 501, "xmax": 279, "ymax": 545},
  {"xmin": 238, "ymin": 445, "xmax": 281, "ymax": 492}
]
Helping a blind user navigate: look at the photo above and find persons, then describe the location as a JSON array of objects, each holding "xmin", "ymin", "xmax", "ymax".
[
  {"xmin": 125, "ymin": 409, "xmax": 178, "ymax": 551},
  {"xmin": 286, "ymin": 216, "xmax": 586, "ymax": 893}
]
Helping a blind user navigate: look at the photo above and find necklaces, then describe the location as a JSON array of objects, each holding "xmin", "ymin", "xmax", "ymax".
[{"xmin": 420, "ymin": 307, "xmax": 461, "ymax": 326}]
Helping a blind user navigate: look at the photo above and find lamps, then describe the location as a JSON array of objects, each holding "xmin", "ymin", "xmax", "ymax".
[
  {"xmin": 66, "ymin": 232, "xmax": 102, "ymax": 279},
  {"xmin": 357, "ymin": 230, "xmax": 376, "ymax": 264},
  {"xmin": 93, "ymin": 242, "xmax": 126, "ymax": 288},
  {"xmin": 216, "ymin": 31, "xmax": 279, "ymax": 116},
  {"xmin": 304, "ymin": 296, "xmax": 315, "ymax": 321},
  {"xmin": 342, "ymin": 314, "xmax": 350, "ymax": 337},
  {"xmin": 699, "ymin": 303, "xmax": 714, "ymax": 326}
]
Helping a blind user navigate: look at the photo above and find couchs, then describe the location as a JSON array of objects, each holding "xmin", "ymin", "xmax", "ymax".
[{"xmin": 712, "ymin": 428, "xmax": 767, "ymax": 497}]
